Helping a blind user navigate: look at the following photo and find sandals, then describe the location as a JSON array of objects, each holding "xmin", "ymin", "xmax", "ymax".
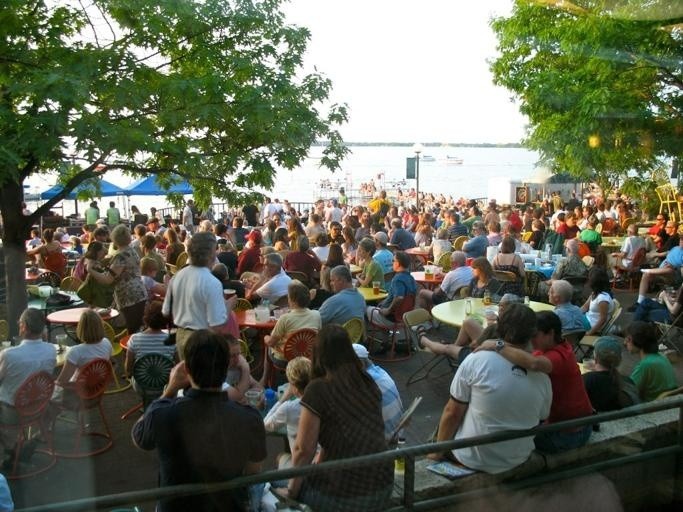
[{"xmin": 417, "ymin": 325, "xmax": 426, "ymax": 349}]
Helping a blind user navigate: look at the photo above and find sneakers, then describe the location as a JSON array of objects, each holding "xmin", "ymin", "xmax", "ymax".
[{"xmin": 375, "ymin": 342, "xmax": 391, "ymax": 354}]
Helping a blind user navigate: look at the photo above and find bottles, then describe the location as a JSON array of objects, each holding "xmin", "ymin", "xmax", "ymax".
[
  {"xmin": 279, "ymin": 390, "xmax": 283, "ymax": 399},
  {"xmin": 393, "ymin": 436, "xmax": 405, "ymax": 475},
  {"xmin": 483, "ymin": 288, "xmax": 491, "ymax": 304},
  {"xmin": 263, "ymin": 389, "xmax": 275, "ymax": 416},
  {"xmin": 536, "ymin": 248, "xmax": 541, "ymax": 265},
  {"xmin": 523, "ymin": 296, "xmax": 530, "ymax": 305},
  {"xmin": 262, "ymin": 297, "xmax": 270, "ymax": 310},
  {"xmin": 548, "ymin": 245, "xmax": 552, "ymax": 260}
]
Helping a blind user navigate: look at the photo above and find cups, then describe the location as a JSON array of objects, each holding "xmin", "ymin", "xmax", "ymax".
[
  {"xmin": 418, "ymin": 239, "xmax": 426, "ymax": 252},
  {"xmin": 50, "ymin": 288, "xmax": 59, "ymax": 295},
  {"xmin": 56, "ymin": 333, "xmax": 67, "ymax": 349},
  {"xmin": 423, "ymin": 265, "xmax": 433, "ymax": 280},
  {"xmin": 434, "ymin": 267, "xmax": 443, "ymax": 279},
  {"xmin": 351, "ymin": 278, "xmax": 356, "ymax": 289},
  {"xmin": 12, "ymin": 336, "xmax": 22, "ymax": 347},
  {"xmin": 273, "ymin": 310, "xmax": 283, "ymax": 320},
  {"xmin": 223, "ymin": 288, "xmax": 236, "ymax": 300},
  {"xmin": 582, "ymin": 359, "xmax": 594, "ymax": 371},
  {"xmin": 431, "ymin": 233, "xmax": 435, "ymax": 240},
  {"xmin": 525, "ymin": 263, "xmax": 531, "ymax": 269},
  {"xmin": 2, "ymin": 341, "xmax": 10, "ymax": 347},
  {"xmin": 76, "ymin": 234, "xmax": 80, "ymax": 238},
  {"xmin": 245, "ymin": 309, "xmax": 255, "ymax": 323},
  {"xmin": 243, "ymin": 279, "xmax": 247, "ymax": 282},
  {"xmin": 535, "ymin": 258, "xmax": 541, "ymax": 267},
  {"xmin": 666, "ymin": 287, "xmax": 674, "ymax": 304},
  {"xmin": 514, "ymin": 252, "xmax": 519, "ymax": 256},
  {"xmin": 245, "ymin": 388, "xmax": 263, "ymax": 407},
  {"xmin": 550, "ymin": 254, "xmax": 562, "ymax": 262},
  {"xmin": 372, "ymin": 281, "xmax": 380, "ymax": 294},
  {"xmin": 464, "ymin": 298, "xmax": 471, "ymax": 314}
]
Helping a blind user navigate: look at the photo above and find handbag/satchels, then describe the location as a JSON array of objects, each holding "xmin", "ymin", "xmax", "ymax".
[{"xmin": 77, "ymin": 274, "xmax": 113, "ymax": 308}]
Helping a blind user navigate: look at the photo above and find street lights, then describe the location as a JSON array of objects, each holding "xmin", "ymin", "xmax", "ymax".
[{"xmin": 412, "ymin": 143, "xmax": 425, "ymax": 209}]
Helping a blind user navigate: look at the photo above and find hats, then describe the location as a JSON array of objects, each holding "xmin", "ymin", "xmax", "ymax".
[
  {"xmin": 372, "ymin": 232, "xmax": 387, "ymax": 244},
  {"xmin": 244, "ymin": 231, "xmax": 261, "ymax": 242},
  {"xmin": 593, "ymin": 336, "xmax": 622, "ymax": 367},
  {"xmin": 352, "ymin": 342, "xmax": 370, "ymax": 359}
]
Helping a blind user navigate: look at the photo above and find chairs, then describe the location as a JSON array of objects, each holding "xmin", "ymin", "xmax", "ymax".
[
  {"xmin": 59, "ymin": 357, "xmax": 114, "ymax": 460},
  {"xmin": 7, "ymin": 370, "xmax": 58, "ymax": 480},
  {"xmin": 7, "ymin": 220, "xmax": 682, "ymax": 417}
]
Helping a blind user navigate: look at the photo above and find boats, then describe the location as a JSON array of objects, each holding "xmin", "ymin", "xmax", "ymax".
[
  {"xmin": 437, "ymin": 154, "xmax": 464, "ymax": 166},
  {"xmin": 417, "ymin": 154, "xmax": 435, "ymax": 163}
]
